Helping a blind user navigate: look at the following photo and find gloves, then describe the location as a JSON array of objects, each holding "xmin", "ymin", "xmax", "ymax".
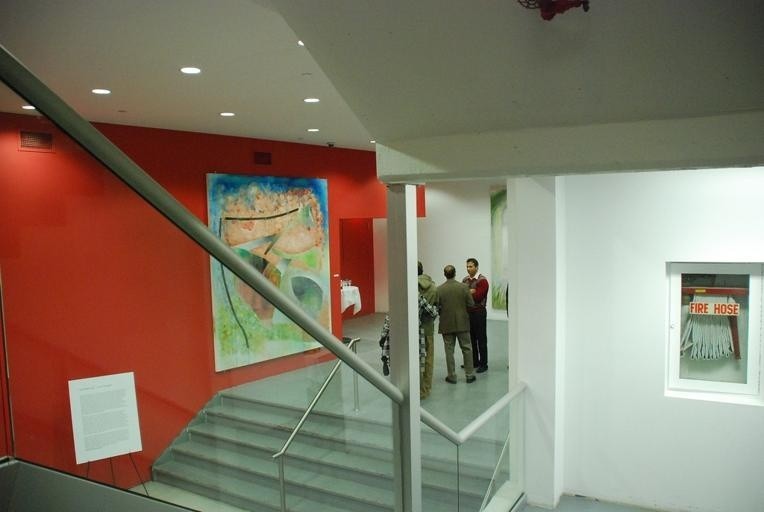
[{"xmin": 381, "ymin": 356, "xmax": 388, "ymax": 361}]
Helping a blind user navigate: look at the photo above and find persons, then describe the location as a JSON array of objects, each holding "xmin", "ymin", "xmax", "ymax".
[
  {"xmin": 436, "ymin": 264, "xmax": 476, "ymax": 383},
  {"xmin": 418, "ymin": 262, "xmax": 439, "ymax": 400},
  {"xmin": 378, "ymin": 291, "xmax": 439, "ymax": 384},
  {"xmin": 460, "ymin": 258, "xmax": 489, "ymax": 372}
]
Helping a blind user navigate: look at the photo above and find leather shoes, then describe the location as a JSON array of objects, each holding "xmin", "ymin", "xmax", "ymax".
[
  {"xmin": 461, "ymin": 365, "xmax": 479, "ymax": 368},
  {"xmin": 477, "ymin": 366, "xmax": 488, "ymax": 373},
  {"xmin": 467, "ymin": 375, "xmax": 476, "ymax": 383},
  {"xmin": 445, "ymin": 377, "xmax": 456, "ymax": 384}
]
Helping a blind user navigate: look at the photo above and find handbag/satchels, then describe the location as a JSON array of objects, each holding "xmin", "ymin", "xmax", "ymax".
[{"xmin": 383, "ymin": 361, "xmax": 389, "ymax": 376}]
